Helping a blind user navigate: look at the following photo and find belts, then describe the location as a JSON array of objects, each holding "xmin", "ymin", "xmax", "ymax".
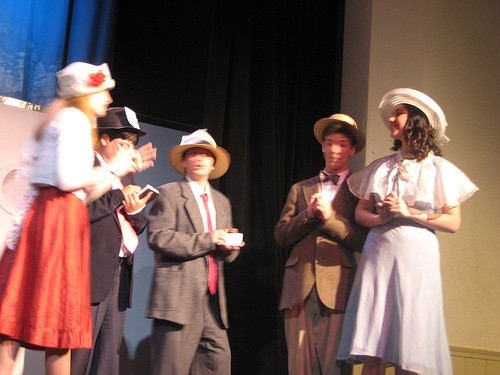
[{"xmin": 119, "ymin": 257, "xmax": 132, "ymax": 265}]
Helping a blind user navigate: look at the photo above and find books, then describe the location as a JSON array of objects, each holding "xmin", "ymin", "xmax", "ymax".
[{"xmin": 119, "ymin": 183, "xmax": 160, "ymax": 218}]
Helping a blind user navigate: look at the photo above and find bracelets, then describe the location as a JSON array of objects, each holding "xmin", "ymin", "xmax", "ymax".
[{"xmin": 109, "ymin": 170, "xmax": 122, "ymax": 180}]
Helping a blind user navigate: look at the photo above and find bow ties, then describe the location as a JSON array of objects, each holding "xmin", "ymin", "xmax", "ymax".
[{"xmin": 319, "ymin": 171, "xmax": 339, "ymax": 185}]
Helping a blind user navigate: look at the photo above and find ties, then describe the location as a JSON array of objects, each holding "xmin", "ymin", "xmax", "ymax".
[{"xmin": 200, "ymin": 193, "xmax": 218, "ymax": 295}]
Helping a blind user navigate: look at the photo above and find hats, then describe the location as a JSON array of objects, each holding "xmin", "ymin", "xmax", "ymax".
[
  {"xmin": 96, "ymin": 107, "xmax": 145, "ymax": 137},
  {"xmin": 378, "ymin": 88, "xmax": 446, "ymax": 144},
  {"xmin": 315, "ymin": 113, "xmax": 366, "ymax": 154},
  {"xmin": 167, "ymin": 129, "xmax": 230, "ymax": 180},
  {"xmin": 57, "ymin": 61, "xmax": 115, "ymax": 100}
]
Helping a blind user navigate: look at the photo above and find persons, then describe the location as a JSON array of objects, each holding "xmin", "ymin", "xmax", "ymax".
[
  {"xmin": 0, "ymin": 61, "xmax": 158, "ymax": 375},
  {"xmin": 273, "ymin": 113, "xmax": 372, "ymax": 375},
  {"xmin": 345, "ymin": 87, "xmax": 481, "ymax": 375},
  {"xmin": 145, "ymin": 128, "xmax": 246, "ymax": 375},
  {"xmin": 69, "ymin": 106, "xmax": 154, "ymax": 374}
]
